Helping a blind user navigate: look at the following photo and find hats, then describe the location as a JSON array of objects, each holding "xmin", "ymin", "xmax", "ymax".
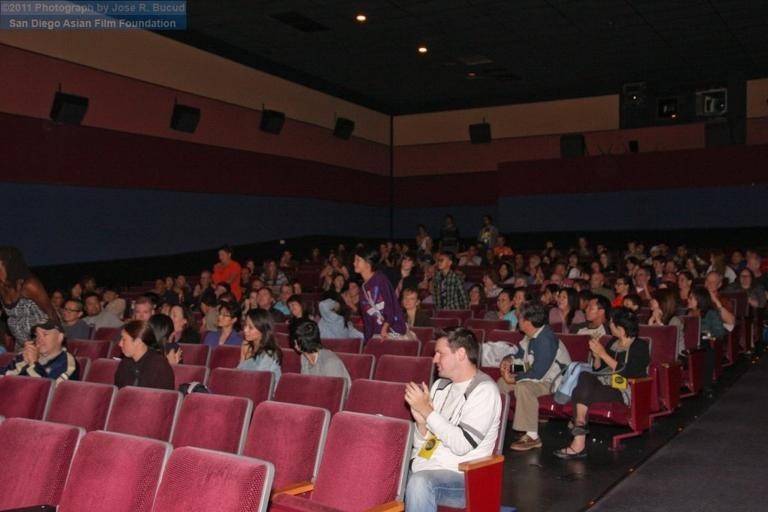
[{"xmin": 31, "ymin": 318, "xmax": 64, "ymax": 334}]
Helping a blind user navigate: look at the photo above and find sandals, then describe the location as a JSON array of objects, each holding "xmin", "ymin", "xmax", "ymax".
[{"xmin": 553, "ymin": 420, "xmax": 588, "ymax": 460}]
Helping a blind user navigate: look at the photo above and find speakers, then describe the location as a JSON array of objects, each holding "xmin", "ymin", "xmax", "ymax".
[
  {"xmin": 168, "ymin": 102, "xmax": 202, "ymax": 135},
  {"xmin": 558, "ymin": 132, "xmax": 587, "ymax": 158},
  {"xmin": 468, "ymin": 122, "xmax": 493, "ymax": 146},
  {"xmin": 49, "ymin": 90, "xmax": 90, "ymax": 125},
  {"xmin": 258, "ymin": 108, "xmax": 286, "ymax": 135},
  {"xmin": 332, "ymin": 117, "xmax": 355, "ymax": 141}
]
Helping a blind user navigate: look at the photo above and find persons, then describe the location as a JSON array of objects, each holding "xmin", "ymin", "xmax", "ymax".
[{"xmin": 0, "ymin": 214, "xmax": 768, "ymax": 512}]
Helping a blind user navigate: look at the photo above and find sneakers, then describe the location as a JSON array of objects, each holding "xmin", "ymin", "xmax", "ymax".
[{"xmin": 510, "ymin": 435, "xmax": 542, "ymax": 450}]
